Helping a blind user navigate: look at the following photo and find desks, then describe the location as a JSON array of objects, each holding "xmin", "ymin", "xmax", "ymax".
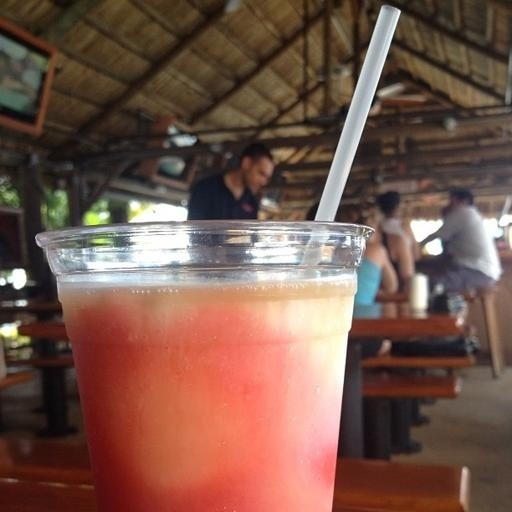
[
  {"xmin": 17, "ymin": 316, "xmax": 465, "ymax": 457},
  {"xmin": 0, "ymin": 441, "xmax": 471, "ymax": 512}
]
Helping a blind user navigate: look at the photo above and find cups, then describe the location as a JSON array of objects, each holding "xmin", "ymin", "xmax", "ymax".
[
  {"xmin": 406, "ymin": 272, "xmax": 430, "ymax": 314},
  {"xmin": 33, "ymin": 218, "xmax": 375, "ymax": 512}
]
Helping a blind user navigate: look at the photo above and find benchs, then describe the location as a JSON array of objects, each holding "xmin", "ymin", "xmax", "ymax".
[
  {"xmin": 64, "ymin": 372, "xmax": 458, "ymax": 461},
  {"xmin": 363, "ymin": 353, "xmax": 471, "ymax": 452}
]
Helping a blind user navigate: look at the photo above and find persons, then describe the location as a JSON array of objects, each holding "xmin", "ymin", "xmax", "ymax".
[
  {"xmin": 186, "ymin": 143, "xmax": 274, "ymax": 283},
  {"xmin": 305, "ymin": 189, "xmax": 503, "ymax": 357}
]
